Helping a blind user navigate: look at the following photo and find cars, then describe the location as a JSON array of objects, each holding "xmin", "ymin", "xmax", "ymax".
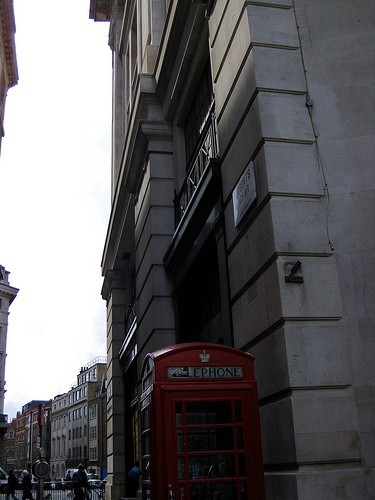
[{"xmin": 87, "ymin": 474, "xmax": 103, "ymax": 488}]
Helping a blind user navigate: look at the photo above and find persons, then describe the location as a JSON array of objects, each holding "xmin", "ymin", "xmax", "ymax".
[
  {"xmin": 6, "ymin": 469, "xmax": 20, "ymax": 500},
  {"xmin": 72, "ymin": 463, "xmax": 87, "ymax": 500},
  {"xmin": 62, "ymin": 470, "xmax": 72, "ymax": 492},
  {"xmin": 21, "ymin": 470, "xmax": 34, "ymax": 500},
  {"xmin": 129, "ymin": 461, "xmax": 140, "ymax": 497}
]
